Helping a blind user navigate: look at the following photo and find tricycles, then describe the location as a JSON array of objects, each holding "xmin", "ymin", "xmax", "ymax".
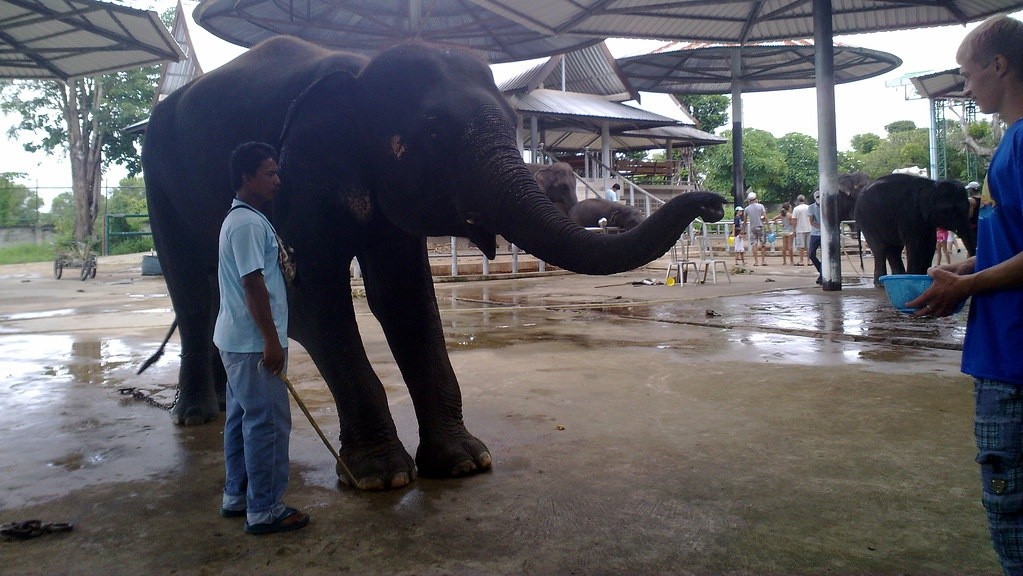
[{"xmin": 53, "ymin": 240, "xmax": 97, "ymax": 280}]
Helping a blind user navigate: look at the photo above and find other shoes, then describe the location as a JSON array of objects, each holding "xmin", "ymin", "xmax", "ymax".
[
  {"xmin": 762, "ymin": 263, "xmax": 768, "ymax": 266},
  {"xmin": 753, "ymin": 264, "xmax": 761, "ymax": 266}
]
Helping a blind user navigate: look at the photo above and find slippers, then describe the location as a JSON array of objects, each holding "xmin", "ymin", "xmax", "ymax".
[
  {"xmin": 244, "ymin": 507, "xmax": 309, "ymax": 535},
  {"xmin": 794, "ymin": 263, "xmax": 804, "ymax": 266},
  {"xmin": 807, "ymin": 263, "xmax": 814, "ymax": 266},
  {"xmin": 221, "ymin": 508, "xmax": 247, "ymax": 518}
]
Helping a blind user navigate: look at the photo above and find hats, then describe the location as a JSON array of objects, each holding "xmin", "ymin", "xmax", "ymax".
[
  {"xmin": 965, "ymin": 182, "xmax": 980, "ymax": 190},
  {"xmin": 747, "ymin": 192, "xmax": 756, "ymax": 200},
  {"xmin": 735, "ymin": 206, "xmax": 745, "ymax": 210},
  {"xmin": 814, "ymin": 191, "xmax": 820, "ymax": 204}
]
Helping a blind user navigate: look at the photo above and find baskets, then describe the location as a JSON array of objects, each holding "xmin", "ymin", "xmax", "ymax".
[{"xmin": 879, "ymin": 271, "xmax": 966, "ymax": 316}]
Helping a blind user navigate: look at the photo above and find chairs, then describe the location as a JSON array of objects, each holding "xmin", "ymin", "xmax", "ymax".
[
  {"xmin": 694, "ymin": 236, "xmax": 732, "ymax": 284},
  {"xmin": 665, "ymin": 238, "xmax": 702, "ymax": 287}
]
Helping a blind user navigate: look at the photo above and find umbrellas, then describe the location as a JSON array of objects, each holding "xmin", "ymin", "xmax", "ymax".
[
  {"xmin": 614, "ymin": 37, "xmax": 902, "ymax": 215},
  {"xmin": 192, "ymin": 0, "xmax": 608, "ymax": 64},
  {"xmin": 473, "ymin": 0, "xmax": 1023, "ymax": 292},
  {"xmin": 0, "ymin": 0, "xmax": 189, "ymax": 84},
  {"xmin": 910, "ymin": 65, "xmax": 975, "ymax": 102},
  {"xmin": 513, "ymin": 125, "xmax": 729, "ymax": 200}
]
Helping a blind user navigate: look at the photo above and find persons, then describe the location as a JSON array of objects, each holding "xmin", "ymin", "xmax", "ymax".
[
  {"xmin": 773, "ymin": 202, "xmax": 796, "ymax": 264},
  {"xmin": 903, "ymin": 13, "xmax": 1023, "ymax": 576},
  {"xmin": 934, "ymin": 228, "xmax": 952, "ymax": 268},
  {"xmin": 743, "ymin": 191, "xmax": 772, "ymax": 266},
  {"xmin": 965, "ymin": 182, "xmax": 981, "ymax": 227},
  {"xmin": 213, "ymin": 141, "xmax": 308, "ymax": 535},
  {"xmin": 605, "ymin": 183, "xmax": 621, "ymax": 203},
  {"xmin": 946, "ymin": 231, "xmax": 962, "ymax": 254},
  {"xmin": 792, "ymin": 194, "xmax": 813, "ymax": 266},
  {"xmin": 734, "ymin": 206, "xmax": 748, "ymax": 265},
  {"xmin": 808, "ymin": 191, "xmax": 823, "ymax": 284}
]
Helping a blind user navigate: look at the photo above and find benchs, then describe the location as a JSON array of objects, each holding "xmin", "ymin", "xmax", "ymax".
[{"xmin": 611, "ymin": 162, "xmax": 677, "ymax": 184}]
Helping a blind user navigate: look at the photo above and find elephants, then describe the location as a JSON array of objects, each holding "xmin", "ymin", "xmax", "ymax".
[
  {"xmin": 835, "ymin": 170, "xmax": 982, "ymax": 289},
  {"xmin": 127, "ymin": 31, "xmax": 735, "ymax": 500}
]
